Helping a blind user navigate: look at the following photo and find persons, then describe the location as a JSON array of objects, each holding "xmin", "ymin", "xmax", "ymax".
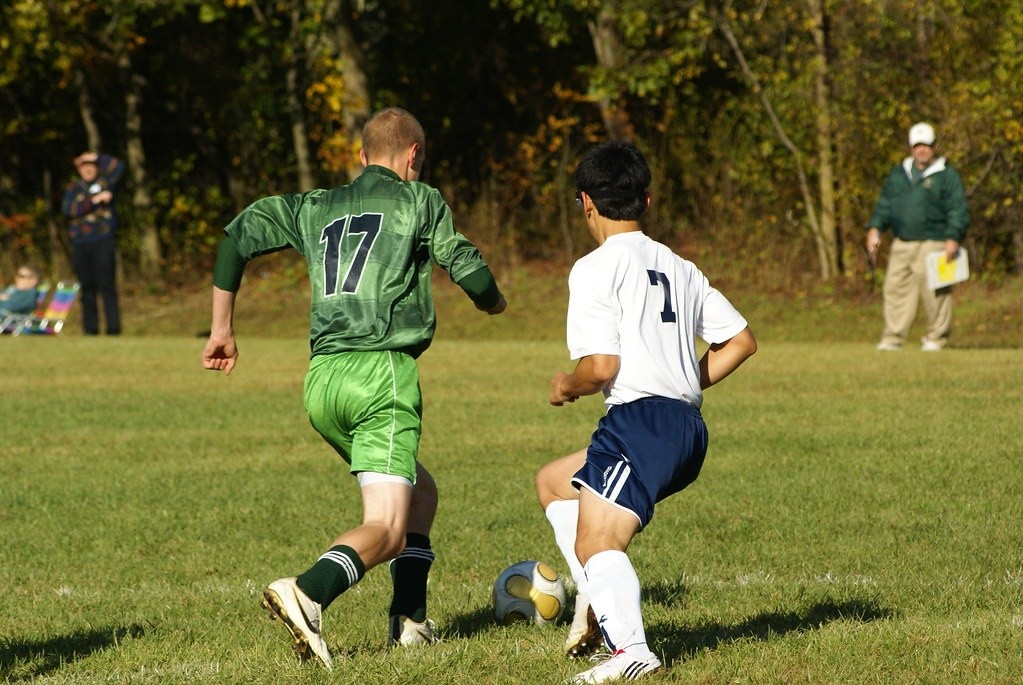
[
  {"xmin": 200, "ymin": 108, "xmax": 508, "ymax": 674},
  {"xmin": 0, "ymin": 265, "xmax": 40, "ymax": 327},
  {"xmin": 60, "ymin": 149, "xmax": 122, "ymax": 337},
  {"xmin": 535, "ymin": 138, "xmax": 759, "ymax": 685},
  {"xmin": 866, "ymin": 122, "xmax": 972, "ymax": 353}
]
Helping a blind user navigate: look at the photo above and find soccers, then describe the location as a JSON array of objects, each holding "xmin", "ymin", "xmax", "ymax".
[{"xmin": 492, "ymin": 558, "xmax": 567, "ymax": 629}]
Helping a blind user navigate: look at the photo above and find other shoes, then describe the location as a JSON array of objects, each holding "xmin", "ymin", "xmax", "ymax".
[
  {"xmin": 877, "ymin": 340, "xmax": 901, "ymax": 351},
  {"xmin": 919, "ymin": 341, "xmax": 939, "ymax": 352}
]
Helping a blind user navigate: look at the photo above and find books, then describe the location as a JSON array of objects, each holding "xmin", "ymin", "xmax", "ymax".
[{"xmin": 926, "ymin": 247, "xmax": 969, "ymax": 290}]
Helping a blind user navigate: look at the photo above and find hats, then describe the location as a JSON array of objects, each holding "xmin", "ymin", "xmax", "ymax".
[{"xmin": 908, "ymin": 121, "xmax": 936, "ymax": 146}]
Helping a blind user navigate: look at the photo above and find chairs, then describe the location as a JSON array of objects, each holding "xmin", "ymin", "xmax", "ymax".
[{"xmin": 1, "ymin": 281, "xmax": 78, "ymax": 337}]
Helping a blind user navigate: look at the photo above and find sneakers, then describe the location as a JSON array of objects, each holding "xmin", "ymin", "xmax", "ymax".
[
  {"xmin": 387, "ymin": 614, "xmax": 436, "ymax": 650},
  {"xmin": 562, "ymin": 649, "xmax": 660, "ymax": 685},
  {"xmin": 261, "ymin": 577, "xmax": 334, "ymax": 675},
  {"xmin": 566, "ymin": 596, "xmax": 606, "ymax": 660}
]
